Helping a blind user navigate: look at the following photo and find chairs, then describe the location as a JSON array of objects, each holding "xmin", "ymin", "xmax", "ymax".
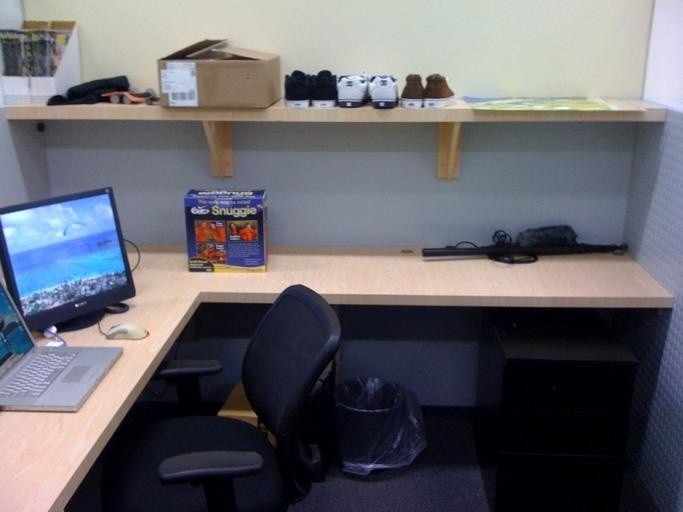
[{"xmin": 102, "ymin": 284, "xmax": 342, "ymax": 511}]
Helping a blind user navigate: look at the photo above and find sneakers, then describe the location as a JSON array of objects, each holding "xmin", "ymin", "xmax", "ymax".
[
  {"xmin": 284, "ymin": 69, "xmax": 310, "ymax": 108},
  {"xmin": 335, "ymin": 72, "xmax": 369, "ymax": 108},
  {"xmin": 309, "ymin": 69, "xmax": 338, "ymax": 108},
  {"xmin": 423, "ymin": 73, "xmax": 458, "ymax": 109},
  {"xmin": 368, "ymin": 72, "xmax": 399, "ymax": 109},
  {"xmin": 401, "ymin": 74, "xmax": 425, "ymax": 109}
]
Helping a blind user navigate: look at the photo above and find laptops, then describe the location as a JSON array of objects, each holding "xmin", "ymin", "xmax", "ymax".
[{"xmin": 0, "ymin": 271, "xmax": 123, "ymax": 412}]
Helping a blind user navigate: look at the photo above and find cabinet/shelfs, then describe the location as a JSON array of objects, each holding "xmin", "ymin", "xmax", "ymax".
[{"xmin": 474, "ymin": 311, "xmax": 637, "ymax": 511}]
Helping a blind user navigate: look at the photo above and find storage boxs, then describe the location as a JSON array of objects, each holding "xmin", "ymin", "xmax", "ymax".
[{"xmin": 155, "ymin": 35, "xmax": 283, "ymax": 110}]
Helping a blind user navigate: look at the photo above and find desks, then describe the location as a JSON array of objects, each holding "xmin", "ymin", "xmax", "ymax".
[{"xmin": 3, "ymin": 242, "xmax": 675, "ymax": 512}]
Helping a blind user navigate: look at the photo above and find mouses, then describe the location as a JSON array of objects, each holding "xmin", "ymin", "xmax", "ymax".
[
  {"xmin": 105, "ymin": 320, "xmax": 149, "ymax": 339},
  {"xmin": 104, "ymin": 302, "xmax": 129, "ymax": 313}
]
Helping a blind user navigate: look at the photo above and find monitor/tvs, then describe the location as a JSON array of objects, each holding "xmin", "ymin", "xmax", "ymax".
[{"xmin": 0, "ymin": 186, "xmax": 136, "ymax": 334}]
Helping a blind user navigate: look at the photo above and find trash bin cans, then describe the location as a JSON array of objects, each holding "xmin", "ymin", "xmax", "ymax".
[{"xmin": 334, "ymin": 377, "xmax": 403, "ymax": 482}]
